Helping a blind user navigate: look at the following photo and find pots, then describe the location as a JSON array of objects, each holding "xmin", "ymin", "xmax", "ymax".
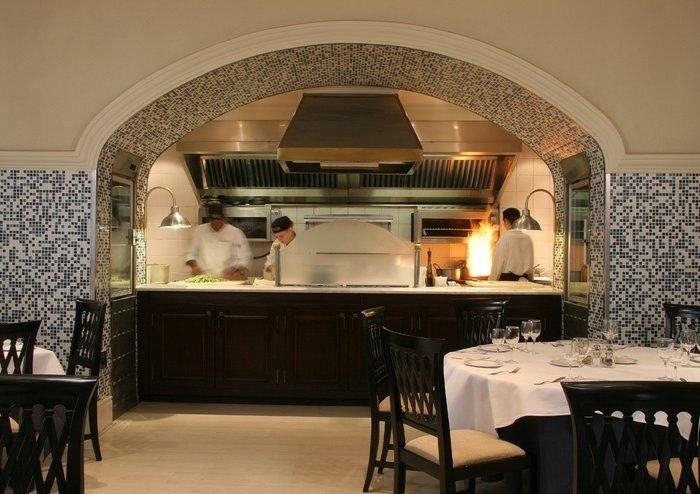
[{"xmin": 434, "ymin": 263, "xmax": 465, "ymax": 280}]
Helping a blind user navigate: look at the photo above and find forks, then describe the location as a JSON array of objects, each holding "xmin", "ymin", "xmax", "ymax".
[
  {"xmin": 487, "ymin": 366, "xmax": 522, "ymax": 376},
  {"xmin": 533, "ymin": 376, "xmax": 568, "ymax": 385}
]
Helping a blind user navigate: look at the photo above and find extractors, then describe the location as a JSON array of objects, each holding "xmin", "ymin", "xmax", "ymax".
[
  {"xmin": 276, "ymin": 93, "xmax": 422, "ymax": 175},
  {"xmin": 176, "ymin": 120, "xmax": 521, "ymax": 203}
]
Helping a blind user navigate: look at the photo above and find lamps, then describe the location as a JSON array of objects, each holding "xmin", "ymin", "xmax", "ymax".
[
  {"xmin": 144, "ymin": 185, "xmax": 192, "ymax": 229},
  {"xmin": 516, "ymin": 188, "xmax": 556, "ymax": 231}
]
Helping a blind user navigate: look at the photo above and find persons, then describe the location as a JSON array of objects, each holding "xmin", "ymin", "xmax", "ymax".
[
  {"xmin": 185, "ymin": 203, "xmax": 253, "ymax": 278},
  {"xmin": 486, "ymin": 207, "xmax": 536, "ymax": 281},
  {"xmin": 265, "ymin": 215, "xmax": 300, "ymax": 279}
]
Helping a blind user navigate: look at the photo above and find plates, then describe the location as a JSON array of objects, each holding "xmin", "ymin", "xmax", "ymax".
[
  {"xmin": 547, "ymin": 359, "xmax": 583, "ymax": 367},
  {"xmin": 613, "ymin": 356, "xmax": 638, "ymax": 365},
  {"xmin": 464, "ymin": 358, "xmax": 507, "ymax": 367},
  {"xmin": 480, "ymin": 344, "xmax": 511, "ymax": 352}
]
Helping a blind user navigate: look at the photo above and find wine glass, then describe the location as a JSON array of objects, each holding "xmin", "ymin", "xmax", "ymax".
[
  {"xmin": 569, "ymin": 320, "xmax": 620, "ymax": 366},
  {"xmin": 656, "ymin": 330, "xmax": 697, "ymax": 380},
  {"xmin": 490, "ymin": 318, "xmax": 542, "ymax": 359}
]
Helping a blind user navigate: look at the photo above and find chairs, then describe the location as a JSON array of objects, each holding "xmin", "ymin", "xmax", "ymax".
[{"xmin": 0, "ymin": 298, "xmax": 106, "ymax": 494}]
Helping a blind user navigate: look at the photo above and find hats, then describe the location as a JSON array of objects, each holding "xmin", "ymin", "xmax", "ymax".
[
  {"xmin": 270, "ymin": 215, "xmax": 295, "ymax": 233},
  {"xmin": 502, "ymin": 207, "xmax": 521, "ymax": 218},
  {"xmin": 206, "ymin": 200, "xmax": 225, "ymax": 216}
]
